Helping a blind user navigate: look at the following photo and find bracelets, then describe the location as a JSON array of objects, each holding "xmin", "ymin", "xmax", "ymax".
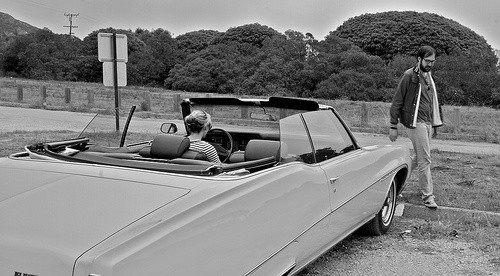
[{"xmin": 390, "ymin": 127, "xmax": 397, "ymax": 130}]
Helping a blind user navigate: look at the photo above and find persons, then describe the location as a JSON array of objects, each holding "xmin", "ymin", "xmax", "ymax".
[
  {"xmin": 389, "ymin": 46, "xmax": 443, "ymax": 208},
  {"xmin": 184, "ymin": 109, "xmax": 221, "ymax": 163}
]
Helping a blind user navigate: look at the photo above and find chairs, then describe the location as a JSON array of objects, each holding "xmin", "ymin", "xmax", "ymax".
[
  {"xmin": 138, "ymin": 133, "xmax": 207, "ymax": 161},
  {"xmin": 228, "ymin": 139, "xmax": 297, "ymax": 163}
]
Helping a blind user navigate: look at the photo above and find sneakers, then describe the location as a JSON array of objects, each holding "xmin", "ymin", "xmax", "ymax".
[{"xmin": 421, "ymin": 192, "xmax": 437, "ymax": 207}]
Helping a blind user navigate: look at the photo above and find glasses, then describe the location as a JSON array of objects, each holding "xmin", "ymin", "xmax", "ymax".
[{"xmin": 423, "ymin": 58, "xmax": 434, "ymax": 63}]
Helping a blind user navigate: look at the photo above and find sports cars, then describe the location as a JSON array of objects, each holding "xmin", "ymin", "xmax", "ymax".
[{"xmin": 0, "ymin": 94, "xmax": 412, "ymax": 276}]
